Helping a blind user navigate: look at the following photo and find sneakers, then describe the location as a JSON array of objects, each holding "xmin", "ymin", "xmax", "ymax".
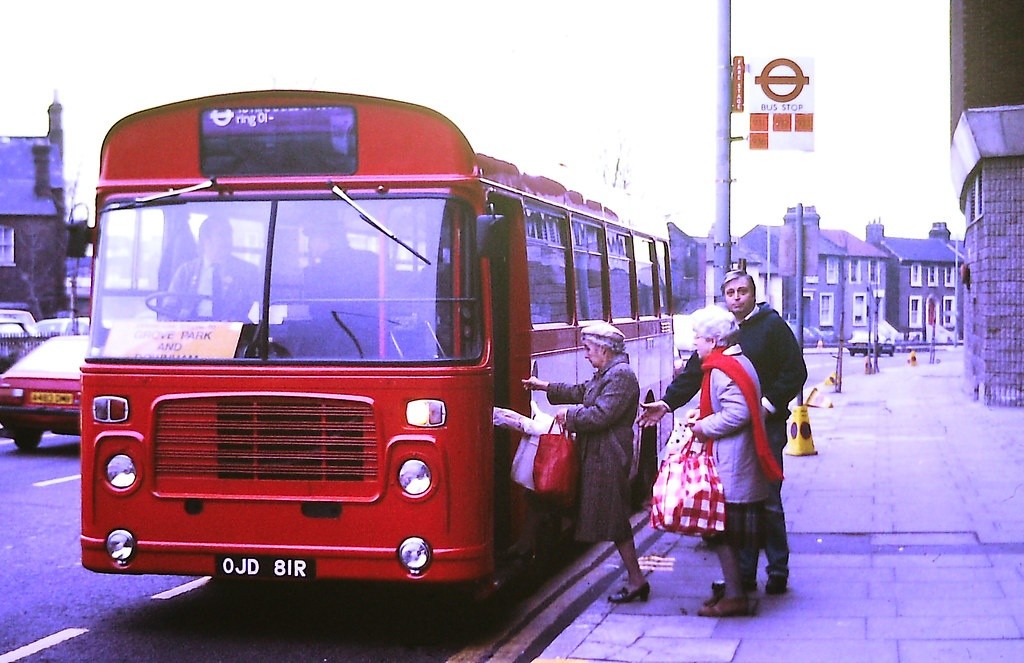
[{"xmin": 698, "ymin": 595, "xmax": 747, "ymax": 617}]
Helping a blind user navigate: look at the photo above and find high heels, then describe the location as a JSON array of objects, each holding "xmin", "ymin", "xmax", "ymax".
[{"xmin": 608, "ymin": 580, "xmax": 650, "ymax": 602}]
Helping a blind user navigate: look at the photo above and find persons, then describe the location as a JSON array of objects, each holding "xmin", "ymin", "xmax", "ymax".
[
  {"xmin": 159, "ymin": 216, "xmax": 258, "ymax": 326},
  {"xmin": 687, "ymin": 305, "xmax": 764, "ymax": 618},
  {"xmin": 495, "ymin": 322, "xmax": 652, "ymax": 603},
  {"xmin": 287, "ymin": 213, "xmax": 397, "ymax": 323},
  {"xmin": 637, "ymin": 269, "xmax": 809, "ymax": 597}
]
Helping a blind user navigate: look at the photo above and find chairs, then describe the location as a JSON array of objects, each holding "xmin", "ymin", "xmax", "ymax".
[{"xmin": 529, "ymin": 261, "xmax": 645, "ymax": 329}]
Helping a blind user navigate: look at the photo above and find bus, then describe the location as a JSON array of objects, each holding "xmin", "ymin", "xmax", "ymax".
[{"xmin": 67, "ymin": 88, "xmax": 677, "ymax": 598}]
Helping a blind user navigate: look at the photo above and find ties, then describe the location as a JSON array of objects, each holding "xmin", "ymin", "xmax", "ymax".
[{"xmin": 211, "ymin": 263, "xmax": 223, "ymax": 321}]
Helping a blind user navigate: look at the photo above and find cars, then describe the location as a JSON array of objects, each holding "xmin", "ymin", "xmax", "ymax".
[
  {"xmin": 0, "ymin": 308, "xmax": 90, "ymax": 363},
  {"xmin": 0, "ymin": 335, "xmax": 89, "ymax": 454},
  {"xmin": 846, "ymin": 331, "xmax": 896, "ymax": 358}
]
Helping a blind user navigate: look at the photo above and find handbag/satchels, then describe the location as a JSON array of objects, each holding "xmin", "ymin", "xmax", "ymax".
[
  {"xmin": 511, "ymin": 414, "xmax": 583, "ymax": 503},
  {"xmin": 648, "ymin": 434, "xmax": 727, "ymax": 537}
]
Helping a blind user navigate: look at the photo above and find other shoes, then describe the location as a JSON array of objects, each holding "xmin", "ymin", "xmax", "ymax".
[
  {"xmin": 712, "ymin": 577, "xmax": 757, "ymax": 592},
  {"xmin": 765, "ymin": 576, "xmax": 787, "ymax": 593}
]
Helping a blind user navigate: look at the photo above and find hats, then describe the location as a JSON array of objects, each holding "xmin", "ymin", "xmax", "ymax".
[
  {"xmin": 581, "ymin": 322, "xmax": 625, "ymax": 342},
  {"xmin": 302, "ymin": 209, "xmax": 346, "ymax": 241}
]
